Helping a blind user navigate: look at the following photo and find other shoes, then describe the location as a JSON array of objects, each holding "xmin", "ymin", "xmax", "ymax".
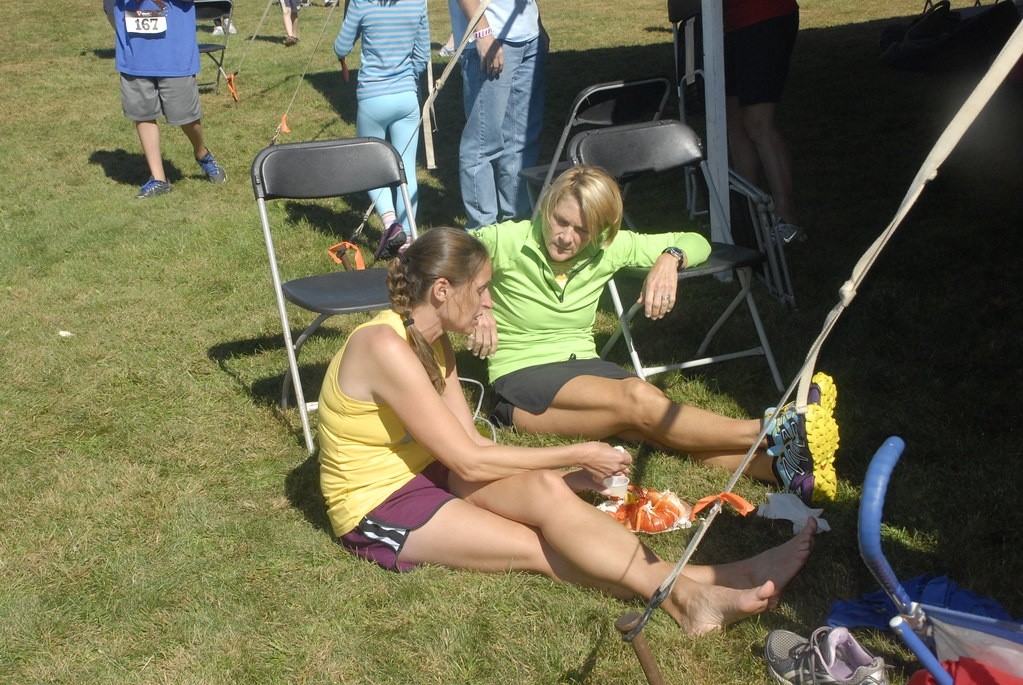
[
  {"xmin": 284, "ymin": 36, "xmax": 299, "ymax": 46},
  {"xmin": 212, "ymin": 26, "xmax": 223, "ymax": 36},
  {"xmin": 223, "ymin": 18, "xmax": 237, "ymax": 34},
  {"xmin": 439, "ymin": 45, "xmax": 454, "ymax": 57}
]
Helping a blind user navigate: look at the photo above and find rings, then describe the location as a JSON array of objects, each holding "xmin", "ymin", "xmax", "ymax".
[{"xmin": 662, "ymin": 298, "xmax": 669, "ymax": 300}]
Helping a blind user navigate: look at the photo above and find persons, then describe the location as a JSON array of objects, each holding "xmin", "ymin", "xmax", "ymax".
[
  {"xmin": 317, "ymin": 227, "xmax": 817, "ymax": 636},
  {"xmin": 332, "ymin": 0, "xmax": 431, "ymax": 249},
  {"xmin": 668, "ymin": 0, "xmax": 800, "ymax": 219},
  {"xmin": 448, "ymin": 0, "xmax": 547, "ymax": 226},
  {"xmin": 212, "ymin": 15, "xmax": 237, "ymax": 36},
  {"xmin": 440, "ymin": 31, "xmax": 455, "ymax": 57},
  {"xmin": 279, "ymin": 0, "xmax": 300, "ymax": 46},
  {"xmin": 465, "ymin": 166, "xmax": 840, "ymax": 505},
  {"xmin": 103, "ymin": 0, "xmax": 224, "ymax": 200}
]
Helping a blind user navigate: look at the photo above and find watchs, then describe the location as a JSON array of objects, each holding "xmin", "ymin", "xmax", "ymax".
[{"xmin": 661, "ymin": 246, "xmax": 683, "ymax": 272}]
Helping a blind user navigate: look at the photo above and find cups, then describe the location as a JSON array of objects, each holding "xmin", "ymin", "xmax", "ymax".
[{"xmin": 603, "ymin": 475, "xmax": 630, "ymax": 498}]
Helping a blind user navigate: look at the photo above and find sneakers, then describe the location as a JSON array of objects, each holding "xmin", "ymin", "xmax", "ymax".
[
  {"xmin": 768, "ymin": 403, "xmax": 840, "ymax": 505},
  {"xmin": 135, "ymin": 175, "xmax": 172, "ymax": 199},
  {"xmin": 762, "ymin": 625, "xmax": 887, "ymax": 685},
  {"xmin": 193, "ymin": 146, "xmax": 227, "ymax": 183},
  {"xmin": 398, "ymin": 236, "xmax": 411, "ymax": 254},
  {"xmin": 765, "ymin": 372, "xmax": 837, "ymax": 444},
  {"xmin": 377, "ymin": 223, "xmax": 407, "ymax": 259}
]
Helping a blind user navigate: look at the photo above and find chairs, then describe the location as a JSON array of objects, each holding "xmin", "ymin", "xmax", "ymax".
[
  {"xmin": 194, "ymin": 0, "xmax": 234, "ymax": 94},
  {"xmin": 728, "ymin": 167, "xmax": 798, "ymax": 311},
  {"xmin": 667, "ymin": 0, "xmax": 706, "ymax": 221},
  {"xmin": 252, "ymin": 136, "xmax": 418, "ymax": 453},
  {"xmin": 518, "ymin": 74, "xmax": 673, "ymax": 232},
  {"xmin": 567, "ymin": 119, "xmax": 785, "ymax": 394}
]
